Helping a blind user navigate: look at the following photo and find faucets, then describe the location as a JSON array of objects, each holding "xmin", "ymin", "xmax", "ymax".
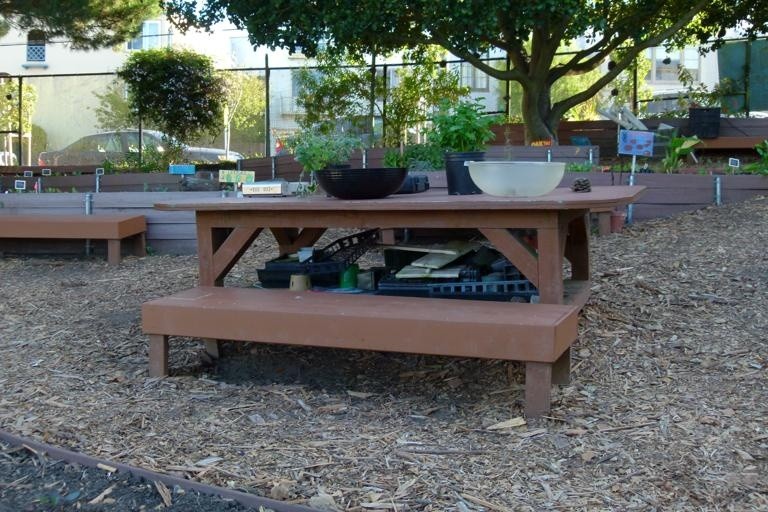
[{"xmin": 358, "ymin": 138, "xmax": 370, "ymax": 165}]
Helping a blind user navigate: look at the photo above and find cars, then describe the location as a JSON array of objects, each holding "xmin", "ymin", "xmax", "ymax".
[
  {"xmin": 598, "ymin": 85, "xmax": 768, "ymax": 122},
  {"xmin": 274, "ymin": 114, "xmax": 438, "ymax": 157},
  {"xmin": 38, "ymin": 129, "xmax": 245, "ymax": 167}
]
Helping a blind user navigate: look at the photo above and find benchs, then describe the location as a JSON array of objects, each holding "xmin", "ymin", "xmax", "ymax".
[
  {"xmin": 1, "ymin": 208, "xmax": 148, "ymax": 268},
  {"xmin": 588, "ymin": 205, "xmax": 614, "ymax": 233},
  {"xmin": 1, "ymin": 99, "xmax": 766, "ymax": 198},
  {"xmin": 138, "ymin": 284, "xmax": 581, "ymax": 427}
]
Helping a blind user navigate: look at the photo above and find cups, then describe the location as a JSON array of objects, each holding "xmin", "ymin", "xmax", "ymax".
[
  {"xmin": 339, "ymin": 264, "xmax": 360, "ymax": 291},
  {"xmin": 289, "ymin": 274, "xmax": 310, "ymax": 290}
]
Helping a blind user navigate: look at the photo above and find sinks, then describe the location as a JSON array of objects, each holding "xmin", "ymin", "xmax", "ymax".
[{"xmin": 317, "ymin": 167, "xmax": 408, "ymax": 199}]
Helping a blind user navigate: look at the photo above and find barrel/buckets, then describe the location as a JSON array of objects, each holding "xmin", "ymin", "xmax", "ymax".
[{"xmin": 689, "ymin": 106, "xmax": 721, "ymax": 140}]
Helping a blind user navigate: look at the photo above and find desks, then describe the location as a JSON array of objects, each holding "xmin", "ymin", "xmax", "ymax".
[{"xmin": 147, "ymin": 183, "xmax": 646, "ymax": 385}]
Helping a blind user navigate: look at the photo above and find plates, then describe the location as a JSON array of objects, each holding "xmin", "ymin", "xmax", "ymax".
[
  {"xmin": 313, "ymin": 164, "xmax": 411, "ymax": 200},
  {"xmin": 462, "ymin": 157, "xmax": 566, "ymax": 198}
]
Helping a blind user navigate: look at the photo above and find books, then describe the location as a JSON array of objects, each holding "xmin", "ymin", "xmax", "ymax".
[{"xmin": 395, "ymin": 241, "xmax": 471, "ymax": 278}]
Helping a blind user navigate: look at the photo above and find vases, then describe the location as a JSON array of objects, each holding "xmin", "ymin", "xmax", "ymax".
[{"xmin": 611, "ymin": 210, "xmax": 627, "ymax": 234}]
